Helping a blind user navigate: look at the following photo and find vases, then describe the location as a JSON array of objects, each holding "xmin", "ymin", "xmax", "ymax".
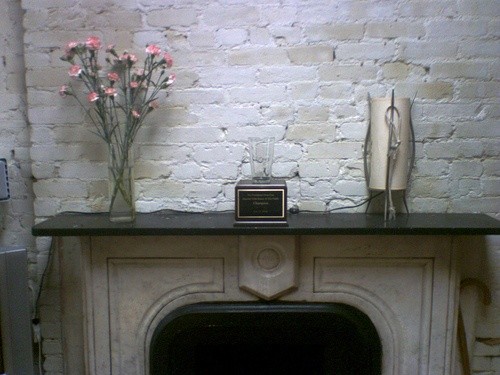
[
  {"xmin": 360, "ymin": 88, "xmax": 422, "ymax": 223},
  {"xmin": 106, "ymin": 142, "xmax": 137, "ymax": 223}
]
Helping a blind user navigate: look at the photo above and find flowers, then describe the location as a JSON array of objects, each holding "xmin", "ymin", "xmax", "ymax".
[{"xmin": 56, "ymin": 36, "xmax": 175, "ymax": 216}]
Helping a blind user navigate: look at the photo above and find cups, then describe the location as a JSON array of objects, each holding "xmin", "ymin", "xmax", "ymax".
[{"xmin": 248, "ymin": 137, "xmax": 274, "ymax": 184}]
